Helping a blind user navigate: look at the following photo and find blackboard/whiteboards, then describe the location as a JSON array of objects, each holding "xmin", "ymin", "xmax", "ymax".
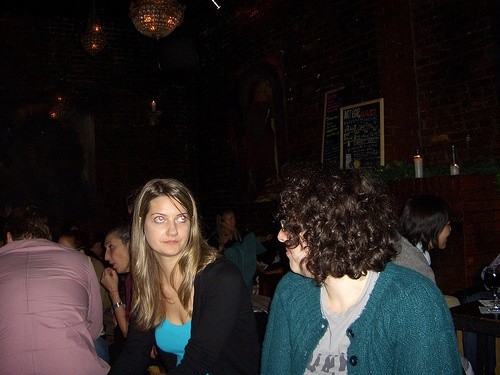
[{"xmin": 322, "ymin": 87, "xmax": 385, "ymax": 167}]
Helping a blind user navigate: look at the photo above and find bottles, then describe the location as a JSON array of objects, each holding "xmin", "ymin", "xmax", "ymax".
[
  {"xmin": 449, "ymin": 145, "xmax": 460, "ymax": 175},
  {"xmin": 413, "ymin": 147, "xmax": 423, "ymax": 179}
]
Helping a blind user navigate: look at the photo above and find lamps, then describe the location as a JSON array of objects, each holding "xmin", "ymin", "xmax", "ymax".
[
  {"xmin": 81, "ymin": 0, "xmax": 109, "ymax": 57},
  {"xmin": 128, "ymin": 0, "xmax": 187, "ymax": 41}
]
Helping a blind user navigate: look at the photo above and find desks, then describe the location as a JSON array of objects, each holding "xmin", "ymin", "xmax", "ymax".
[
  {"xmin": 257, "ymin": 267, "xmax": 288, "ymax": 329},
  {"xmin": 450, "ymin": 297, "xmax": 500, "ymax": 375}
]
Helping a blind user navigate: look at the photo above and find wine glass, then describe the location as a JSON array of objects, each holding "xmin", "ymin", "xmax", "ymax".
[{"xmin": 484, "ymin": 267, "xmax": 500, "ymax": 310}]
